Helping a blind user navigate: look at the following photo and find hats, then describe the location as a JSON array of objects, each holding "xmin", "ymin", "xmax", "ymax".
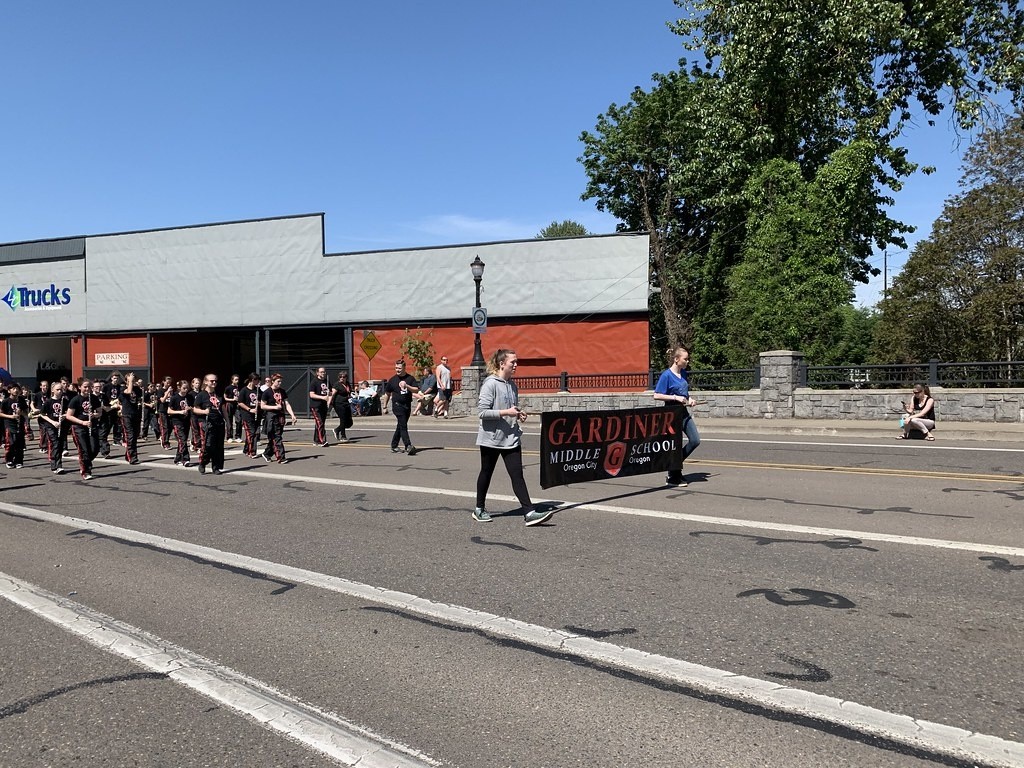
[{"xmin": 359, "ymin": 380, "xmax": 368, "ymax": 384}]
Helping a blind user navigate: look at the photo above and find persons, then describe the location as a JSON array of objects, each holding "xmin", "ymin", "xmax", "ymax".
[
  {"xmin": 412, "ymin": 367, "xmax": 435, "ymax": 416},
  {"xmin": 309, "ymin": 368, "xmax": 333, "ymax": 446},
  {"xmin": 383, "ymin": 360, "xmax": 419, "ymax": 455},
  {"xmin": 1, "ymin": 371, "xmax": 268, "ymax": 473},
  {"xmin": 434, "ymin": 384, "xmax": 449, "ymax": 419},
  {"xmin": 353, "ymin": 381, "xmax": 376, "ymax": 415},
  {"xmin": 328, "ymin": 372, "xmax": 353, "ymax": 442},
  {"xmin": 192, "ymin": 374, "xmax": 230, "ymax": 475},
  {"xmin": 67, "ymin": 377, "xmax": 102, "ymax": 479},
  {"xmin": 472, "ymin": 349, "xmax": 553, "ymax": 526},
  {"xmin": 434, "ymin": 355, "xmax": 451, "ymax": 420},
  {"xmin": 654, "ymin": 348, "xmax": 700, "ymax": 486},
  {"xmin": 261, "ymin": 373, "xmax": 297, "ymax": 464},
  {"xmin": 895, "ymin": 384, "xmax": 936, "ymax": 441}
]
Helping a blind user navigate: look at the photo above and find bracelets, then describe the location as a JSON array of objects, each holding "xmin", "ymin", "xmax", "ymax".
[
  {"xmin": 383, "ymin": 407, "xmax": 387, "ymax": 409},
  {"xmin": 909, "ymin": 416, "xmax": 912, "ymax": 421}
]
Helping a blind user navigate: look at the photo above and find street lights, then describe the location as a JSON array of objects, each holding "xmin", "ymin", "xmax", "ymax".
[{"xmin": 469, "ymin": 254, "xmax": 489, "ymax": 365}]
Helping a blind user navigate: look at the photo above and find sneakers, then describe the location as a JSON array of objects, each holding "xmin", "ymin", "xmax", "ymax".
[
  {"xmin": 525, "ymin": 511, "xmax": 553, "ymax": 526},
  {"xmin": 408, "ymin": 445, "xmax": 417, "ymax": 455},
  {"xmin": 391, "ymin": 447, "xmax": 405, "ymax": 453},
  {"xmin": 665, "ymin": 476, "xmax": 687, "ymax": 486},
  {"xmin": 333, "ymin": 429, "xmax": 339, "ymax": 440},
  {"xmin": 471, "ymin": 509, "xmax": 492, "ymax": 522},
  {"xmin": 340, "ymin": 436, "xmax": 350, "ymax": 443}
]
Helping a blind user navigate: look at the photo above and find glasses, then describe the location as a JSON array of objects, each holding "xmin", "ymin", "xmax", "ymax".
[
  {"xmin": 441, "ymin": 359, "xmax": 449, "ymax": 361},
  {"xmin": 265, "ymin": 380, "xmax": 270, "ymax": 382},
  {"xmin": 339, "ymin": 375, "xmax": 346, "ymax": 378},
  {"xmin": 209, "ymin": 380, "xmax": 217, "ymax": 382},
  {"xmin": 913, "ymin": 390, "xmax": 923, "ymax": 394}
]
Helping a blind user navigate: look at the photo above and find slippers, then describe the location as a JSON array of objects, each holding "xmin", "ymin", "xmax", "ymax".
[
  {"xmin": 895, "ymin": 435, "xmax": 909, "ymax": 440},
  {"xmin": 927, "ymin": 437, "xmax": 935, "ymax": 441}
]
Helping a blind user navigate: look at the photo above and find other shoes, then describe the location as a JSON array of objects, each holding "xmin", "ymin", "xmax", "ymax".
[
  {"xmin": 434, "ymin": 408, "xmax": 438, "ymax": 418},
  {"xmin": 313, "ymin": 442, "xmax": 320, "ymax": 445},
  {"xmin": 444, "ymin": 415, "xmax": 448, "ymax": 419},
  {"xmin": 0, "ymin": 429, "xmax": 290, "ymax": 479},
  {"xmin": 318, "ymin": 442, "xmax": 329, "ymax": 447}
]
